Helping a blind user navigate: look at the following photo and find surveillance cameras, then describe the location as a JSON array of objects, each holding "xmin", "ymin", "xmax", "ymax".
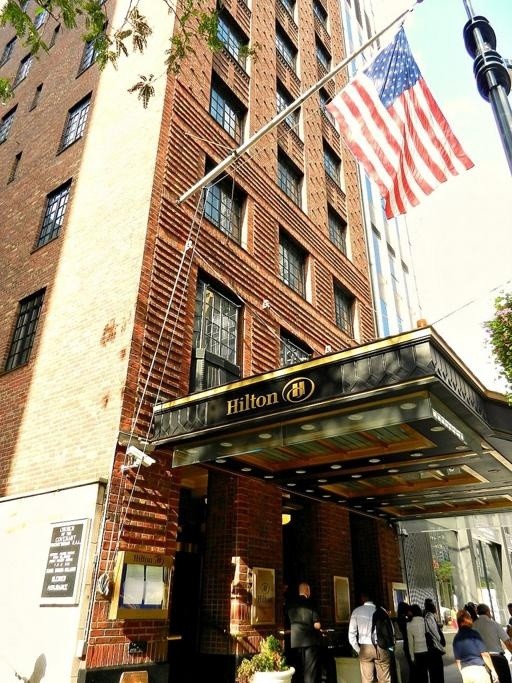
[
  {"xmin": 125, "ymin": 445, "xmax": 157, "ymax": 468},
  {"xmin": 401, "ymin": 528, "xmax": 409, "ymax": 538}
]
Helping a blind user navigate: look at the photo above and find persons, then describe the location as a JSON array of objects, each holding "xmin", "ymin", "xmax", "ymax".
[
  {"xmin": 396, "ymin": 598, "xmax": 511, "ymax": 683},
  {"xmin": 282, "ymin": 581, "xmax": 289, "ymax": 629},
  {"xmin": 288, "ymin": 582, "xmax": 322, "ymax": 682},
  {"xmin": 348, "ymin": 588, "xmax": 396, "ymax": 683}
]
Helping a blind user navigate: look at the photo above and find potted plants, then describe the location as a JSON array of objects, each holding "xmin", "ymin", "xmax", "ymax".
[{"xmin": 234, "ymin": 635, "xmax": 295, "ymax": 682}]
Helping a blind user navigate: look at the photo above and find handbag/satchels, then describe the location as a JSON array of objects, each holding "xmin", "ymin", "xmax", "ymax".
[{"xmin": 423, "ymin": 619, "xmax": 446, "ymax": 655}]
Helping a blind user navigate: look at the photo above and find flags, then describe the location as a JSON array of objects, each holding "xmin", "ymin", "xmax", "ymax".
[{"xmin": 323, "ymin": 16, "xmax": 474, "ymax": 221}]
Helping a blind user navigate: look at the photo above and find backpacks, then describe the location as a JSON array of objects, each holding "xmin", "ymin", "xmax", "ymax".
[{"xmin": 370, "ymin": 606, "xmax": 394, "ymax": 649}]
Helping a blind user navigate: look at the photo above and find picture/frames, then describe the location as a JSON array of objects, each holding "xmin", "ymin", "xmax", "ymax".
[
  {"xmin": 36, "ymin": 515, "xmax": 91, "ymax": 606},
  {"xmin": 251, "ymin": 566, "xmax": 275, "ymax": 626},
  {"xmin": 332, "ymin": 575, "xmax": 351, "ymax": 623}
]
